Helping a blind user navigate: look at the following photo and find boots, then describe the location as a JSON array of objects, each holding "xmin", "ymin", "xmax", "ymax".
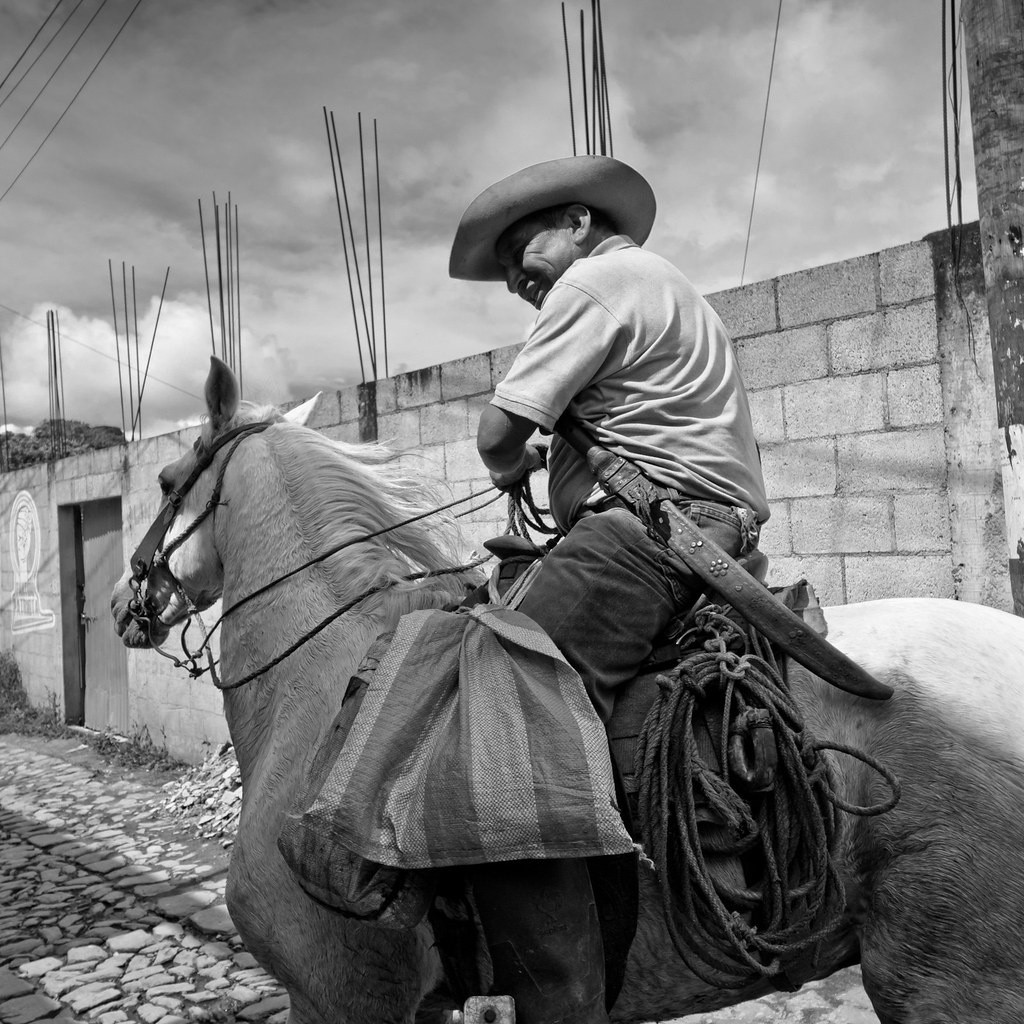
[{"xmin": 464, "ymin": 858, "xmax": 606, "ymax": 1024}]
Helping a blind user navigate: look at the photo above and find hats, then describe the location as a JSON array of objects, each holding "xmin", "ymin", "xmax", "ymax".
[{"xmin": 449, "ymin": 155, "xmax": 656, "ymax": 282}]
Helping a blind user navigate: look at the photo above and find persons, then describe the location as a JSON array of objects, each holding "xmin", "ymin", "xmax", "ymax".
[{"xmin": 449, "ymin": 156, "xmax": 771, "ymax": 1024}]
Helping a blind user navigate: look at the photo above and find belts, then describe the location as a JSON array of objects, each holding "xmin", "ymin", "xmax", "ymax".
[{"xmin": 593, "ymin": 489, "xmax": 703, "ymax": 514}]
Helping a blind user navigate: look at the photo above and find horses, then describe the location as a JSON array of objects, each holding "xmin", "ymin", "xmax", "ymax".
[{"xmin": 108, "ymin": 351, "xmax": 1023, "ymax": 1024}]
